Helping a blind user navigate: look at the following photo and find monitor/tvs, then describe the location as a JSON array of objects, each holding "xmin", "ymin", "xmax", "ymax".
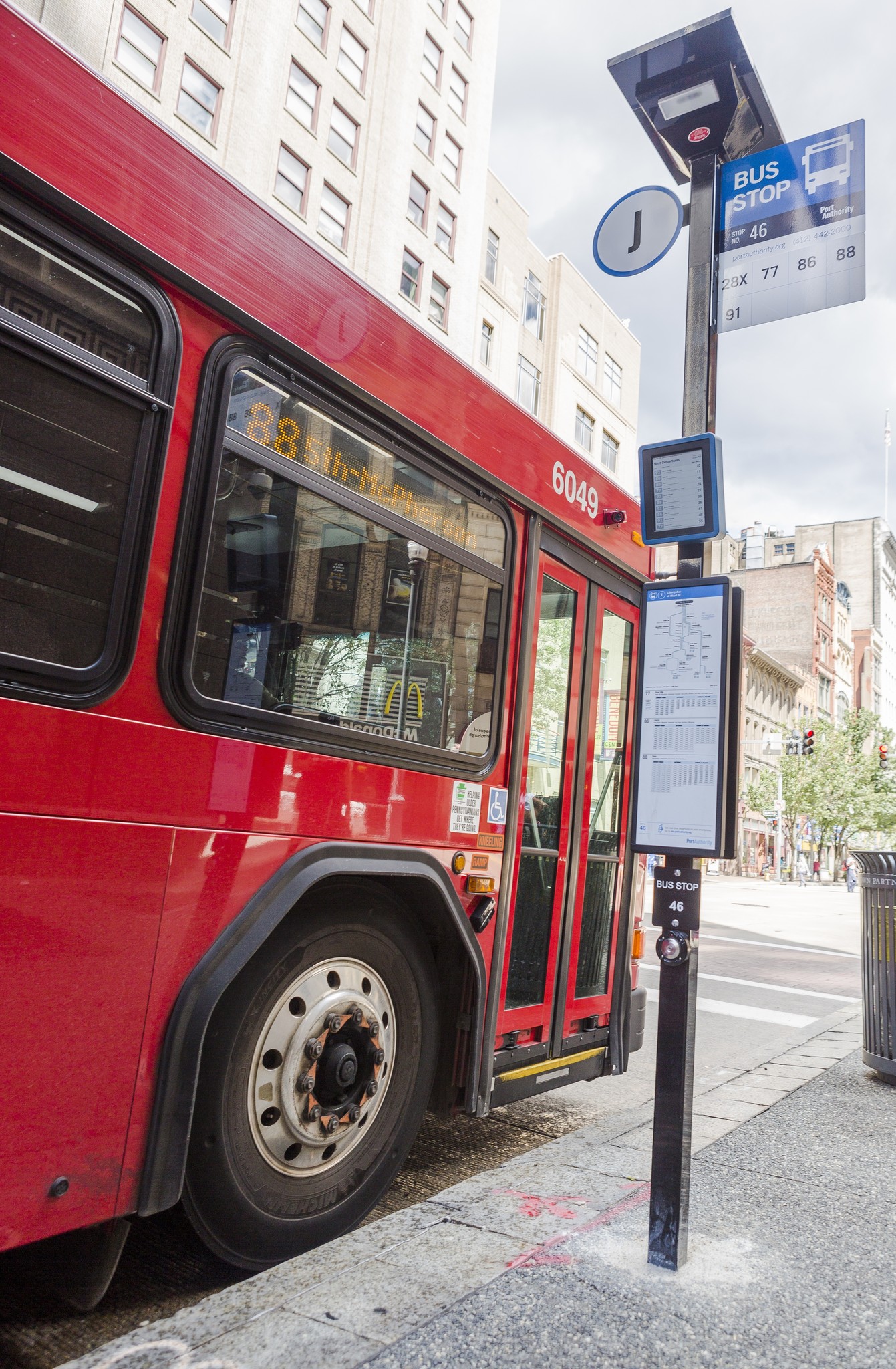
[{"xmin": 635, "ymin": 432, "xmax": 726, "ymax": 548}]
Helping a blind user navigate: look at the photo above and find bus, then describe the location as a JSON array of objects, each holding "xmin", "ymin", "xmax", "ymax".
[{"xmin": 2, "ymin": 0, "xmax": 662, "ymax": 1314}]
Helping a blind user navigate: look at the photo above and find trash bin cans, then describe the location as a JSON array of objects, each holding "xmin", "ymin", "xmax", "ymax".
[
  {"xmin": 506, "ymin": 791, "xmax": 619, "ymax": 1004},
  {"xmin": 847, "ymin": 846, "xmax": 896, "ymax": 1087}
]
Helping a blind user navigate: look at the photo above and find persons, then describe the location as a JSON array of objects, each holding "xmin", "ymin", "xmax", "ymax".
[
  {"xmin": 846, "ymin": 855, "xmax": 857, "ymax": 893},
  {"xmin": 766, "ymin": 852, "xmax": 773, "ymax": 866},
  {"xmin": 811, "ymin": 858, "xmax": 820, "ymax": 882},
  {"xmin": 781, "ymin": 857, "xmax": 786, "ymax": 865},
  {"xmin": 797, "ymin": 857, "xmax": 810, "ymax": 887}
]
